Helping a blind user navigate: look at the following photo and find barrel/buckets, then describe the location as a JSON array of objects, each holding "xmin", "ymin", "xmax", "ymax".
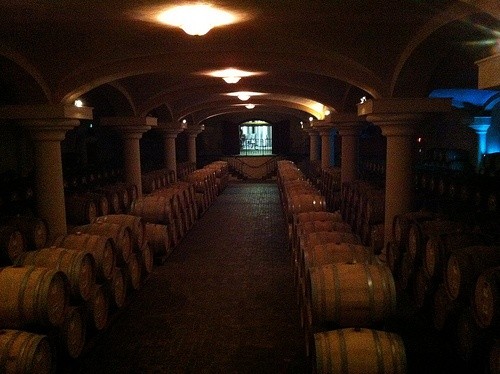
[
  {"xmin": 277, "ymin": 148, "xmax": 499, "ymax": 373},
  {"xmin": 0, "ymin": 157, "xmax": 229, "ymax": 374}
]
